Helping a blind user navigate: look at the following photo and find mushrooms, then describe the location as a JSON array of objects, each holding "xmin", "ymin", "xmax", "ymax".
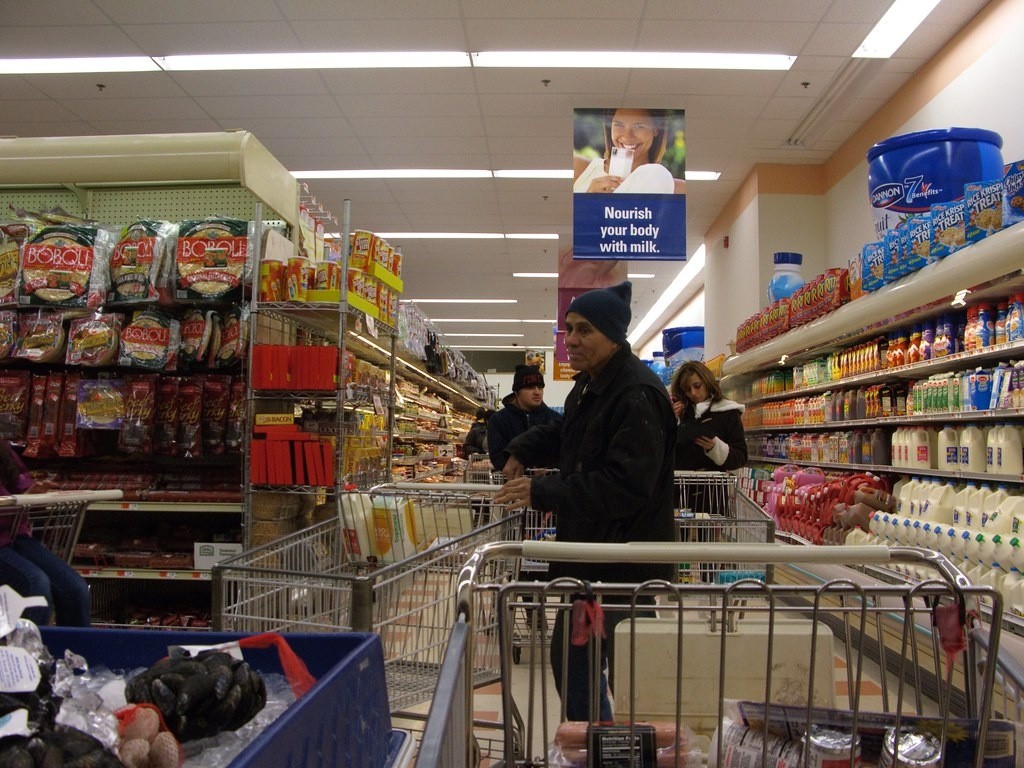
[{"xmin": 121, "ymin": 706, "xmax": 181, "ymax": 768}]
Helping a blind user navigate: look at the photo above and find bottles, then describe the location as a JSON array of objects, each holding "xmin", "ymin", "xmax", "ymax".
[
  {"xmin": 339, "ymin": 481, "xmax": 414, "ymax": 563},
  {"xmin": 762, "ymin": 294, "xmax": 1024, "ymax": 615}
]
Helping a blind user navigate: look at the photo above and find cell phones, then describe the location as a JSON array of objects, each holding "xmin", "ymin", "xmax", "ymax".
[{"xmin": 700, "ymin": 417, "xmax": 713, "ymax": 440}]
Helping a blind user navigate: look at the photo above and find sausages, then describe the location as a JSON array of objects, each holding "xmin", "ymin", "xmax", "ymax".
[{"xmin": 554, "ymin": 719, "xmax": 689, "ymax": 768}]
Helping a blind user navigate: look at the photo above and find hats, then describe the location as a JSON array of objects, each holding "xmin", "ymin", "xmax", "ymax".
[
  {"xmin": 565, "ymin": 281, "xmax": 632, "ymax": 346},
  {"xmin": 512, "ymin": 364, "xmax": 545, "ymax": 392}
]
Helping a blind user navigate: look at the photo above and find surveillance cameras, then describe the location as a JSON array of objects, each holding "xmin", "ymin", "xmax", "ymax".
[{"xmin": 512, "ymin": 344, "xmax": 518, "ymax": 346}]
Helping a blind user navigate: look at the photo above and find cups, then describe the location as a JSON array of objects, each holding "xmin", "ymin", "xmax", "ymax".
[{"xmin": 609, "ymin": 147, "xmax": 634, "ymax": 179}]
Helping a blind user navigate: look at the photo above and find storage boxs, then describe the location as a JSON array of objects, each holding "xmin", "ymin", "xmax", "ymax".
[
  {"xmin": 0, "ymin": 626, "xmax": 392, "ymax": 768},
  {"xmin": 612, "ymin": 618, "xmax": 838, "ymax": 740},
  {"xmin": 192, "ymin": 259, "xmax": 404, "ymax": 572}
]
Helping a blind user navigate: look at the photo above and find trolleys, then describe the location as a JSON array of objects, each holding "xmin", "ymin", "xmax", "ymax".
[
  {"xmin": 467, "ymin": 453, "xmax": 496, "ymax": 534},
  {"xmin": 208, "ymin": 481, "xmax": 525, "ymax": 768},
  {"xmin": 413, "ymin": 544, "xmax": 1024, "ymax": 768},
  {"xmin": 490, "ymin": 466, "xmax": 564, "ymax": 664},
  {"xmin": 673, "ymin": 471, "xmax": 776, "ymax": 632},
  {"xmin": 0, "ymin": 489, "xmax": 123, "ymax": 565}
]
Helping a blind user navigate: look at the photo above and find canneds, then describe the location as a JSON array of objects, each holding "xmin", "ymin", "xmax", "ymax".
[
  {"xmin": 800, "ymin": 723, "xmax": 944, "ymax": 768},
  {"xmin": 259, "ymin": 229, "xmax": 404, "ymax": 303}
]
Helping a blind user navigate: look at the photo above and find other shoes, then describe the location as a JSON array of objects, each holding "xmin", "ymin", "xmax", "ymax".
[
  {"xmin": 473, "ymin": 514, "xmax": 489, "ymax": 528},
  {"xmin": 526, "ymin": 607, "xmax": 549, "ymax": 632}
]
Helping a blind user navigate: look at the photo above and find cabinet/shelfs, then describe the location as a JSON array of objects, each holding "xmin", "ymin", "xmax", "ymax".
[
  {"xmin": 237, "ymin": 197, "xmax": 400, "ymax": 563},
  {"xmin": 724, "ymin": 220, "xmax": 1024, "ymax": 724},
  {"xmin": 0, "ymin": 131, "xmax": 302, "ymax": 634},
  {"xmin": 294, "ymin": 331, "xmax": 488, "ymax": 496}
]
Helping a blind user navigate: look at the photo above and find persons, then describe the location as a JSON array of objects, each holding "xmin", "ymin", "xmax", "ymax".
[
  {"xmin": 575, "ymin": 109, "xmax": 686, "ymax": 195},
  {"xmin": 464, "ymin": 364, "xmax": 563, "ymax": 628},
  {"xmin": 671, "ymin": 358, "xmax": 750, "ymax": 581},
  {"xmin": 0, "ymin": 434, "xmax": 91, "ymax": 627},
  {"xmin": 493, "ymin": 280, "xmax": 678, "ymax": 721}
]
